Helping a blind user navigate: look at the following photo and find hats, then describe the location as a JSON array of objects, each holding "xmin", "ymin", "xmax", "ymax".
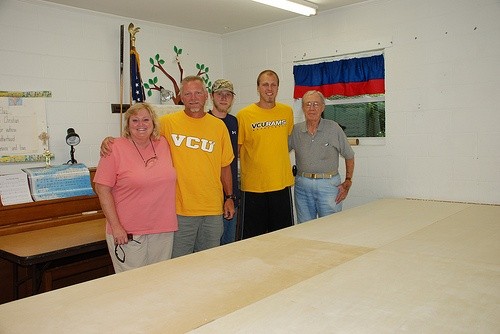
[{"xmin": 212, "ymin": 79, "xmax": 236, "ymax": 95}]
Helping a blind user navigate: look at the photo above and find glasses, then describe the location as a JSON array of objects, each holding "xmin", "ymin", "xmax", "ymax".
[
  {"xmin": 115, "ymin": 239, "xmax": 140, "ymax": 263},
  {"xmin": 304, "ymin": 102, "xmax": 320, "ymax": 107}
]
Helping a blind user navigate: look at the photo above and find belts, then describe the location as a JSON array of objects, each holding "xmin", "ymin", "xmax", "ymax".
[{"xmin": 297, "ymin": 170, "xmax": 338, "ymax": 178}]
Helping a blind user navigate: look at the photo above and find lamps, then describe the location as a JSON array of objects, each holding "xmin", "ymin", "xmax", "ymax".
[
  {"xmin": 160, "ymin": 86, "xmax": 174, "ymax": 105},
  {"xmin": 66, "ymin": 128, "xmax": 81, "ymax": 164},
  {"xmin": 253, "ymin": 0, "xmax": 318, "ymax": 17}
]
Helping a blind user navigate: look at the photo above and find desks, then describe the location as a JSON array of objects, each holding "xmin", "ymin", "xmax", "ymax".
[{"xmin": 0, "ymin": 217, "xmax": 107, "ymax": 300}]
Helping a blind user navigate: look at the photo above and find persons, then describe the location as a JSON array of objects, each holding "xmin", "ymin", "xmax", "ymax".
[
  {"xmin": 286, "ymin": 91, "xmax": 354, "ymax": 226},
  {"xmin": 93, "ymin": 103, "xmax": 179, "ymax": 274},
  {"xmin": 99, "ymin": 75, "xmax": 235, "ymax": 259},
  {"xmin": 235, "ymin": 70, "xmax": 294, "ymax": 240},
  {"xmin": 205, "ymin": 78, "xmax": 238, "ymax": 245}
]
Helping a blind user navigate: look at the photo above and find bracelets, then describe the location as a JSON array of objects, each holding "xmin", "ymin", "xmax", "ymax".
[
  {"xmin": 224, "ymin": 194, "xmax": 236, "ymax": 201},
  {"xmin": 345, "ymin": 177, "xmax": 351, "ymax": 181}
]
